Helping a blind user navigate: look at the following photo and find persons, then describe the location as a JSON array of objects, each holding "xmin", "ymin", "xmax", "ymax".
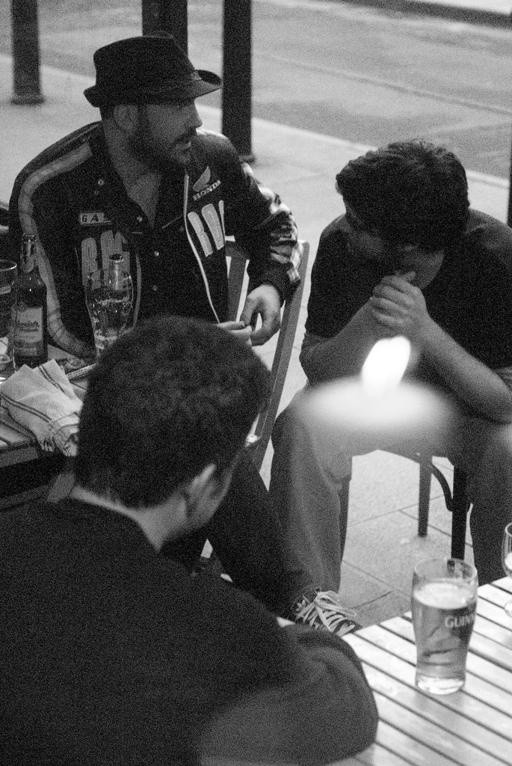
[
  {"xmin": 9, "ymin": 29, "xmax": 367, "ymax": 636},
  {"xmin": 2, "ymin": 314, "xmax": 376, "ymax": 764},
  {"xmin": 267, "ymin": 142, "xmax": 512, "ymax": 585}
]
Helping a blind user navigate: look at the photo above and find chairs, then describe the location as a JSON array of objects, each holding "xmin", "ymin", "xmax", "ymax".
[
  {"xmin": 195, "ymin": 241, "xmax": 309, "ymax": 577},
  {"xmin": 339, "ymin": 440, "xmax": 473, "ymax": 565}
]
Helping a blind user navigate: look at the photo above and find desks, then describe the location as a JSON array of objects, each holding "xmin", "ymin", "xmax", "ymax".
[
  {"xmin": 0, "ymin": 343, "xmax": 91, "ymax": 511},
  {"xmin": 327, "ymin": 574, "xmax": 512, "ymax": 765}
]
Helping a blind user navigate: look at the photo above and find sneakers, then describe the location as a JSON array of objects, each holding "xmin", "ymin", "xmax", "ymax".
[{"xmin": 288, "ymin": 590, "xmax": 362, "ymax": 638}]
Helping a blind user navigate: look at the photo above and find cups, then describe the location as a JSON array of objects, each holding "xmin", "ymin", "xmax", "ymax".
[
  {"xmin": 497, "ymin": 523, "xmax": 512, "ymax": 617},
  {"xmin": 406, "ymin": 554, "xmax": 484, "ymax": 698},
  {"xmin": 0, "ymin": 255, "xmax": 16, "ymax": 371}
]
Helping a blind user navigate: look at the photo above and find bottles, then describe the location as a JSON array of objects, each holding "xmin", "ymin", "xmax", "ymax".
[
  {"xmin": 107, "ymin": 253, "xmax": 129, "ymax": 295},
  {"xmin": 6, "ymin": 230, "xmax": 51, "ymax": 367}
]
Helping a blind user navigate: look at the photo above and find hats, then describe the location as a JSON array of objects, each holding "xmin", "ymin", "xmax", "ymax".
[{"xmin": 85, "ymin": 30, "xmax": 224, "ymax": 102}]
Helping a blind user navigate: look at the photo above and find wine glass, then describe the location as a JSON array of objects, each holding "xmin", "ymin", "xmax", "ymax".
[{"xmin": 84, "ymin": 270, "xmax": 140, "ymax": 354}]
